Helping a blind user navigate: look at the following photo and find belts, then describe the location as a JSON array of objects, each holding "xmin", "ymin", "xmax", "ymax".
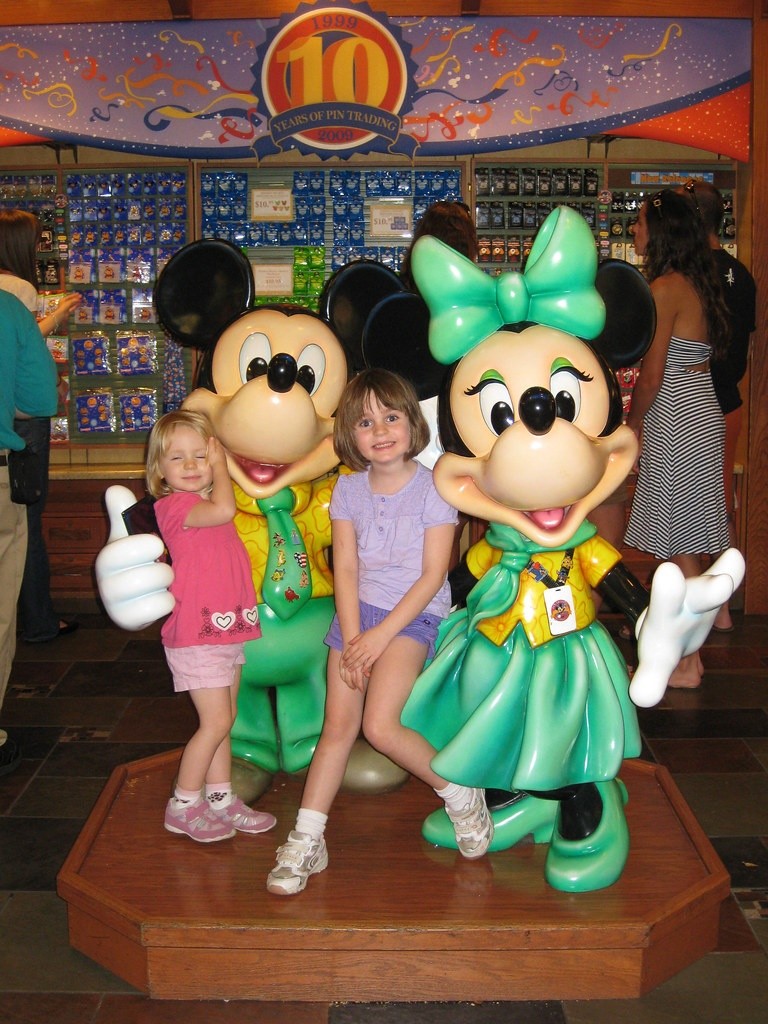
[{"xmin": 0, "ymin": 455, "xmax": 9, "ymax": 466}]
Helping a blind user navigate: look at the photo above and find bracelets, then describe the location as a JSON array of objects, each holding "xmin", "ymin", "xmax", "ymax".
[{"xmin": 50, "ymin": 312, "xmax": 59, "ymax": 335}]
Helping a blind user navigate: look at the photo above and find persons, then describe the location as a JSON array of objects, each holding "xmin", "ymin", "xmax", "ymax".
[
  {"xmin": 618, "ymin": 181, "xmax": 757, "ymax": 688},
  {"xmin": 265, "ymin": 369, "xmax": 494, "ymax": 895},
  {"xmin": 147, "ymin": 410, "xmax": 277, "ymax": 842},
  {"xmin": 0, "ymin": 207, "xmax": 83, "ymax": 743},
  {"xmin": 398, "ymin": 203, "xmax": 477, "ymax": 294}
]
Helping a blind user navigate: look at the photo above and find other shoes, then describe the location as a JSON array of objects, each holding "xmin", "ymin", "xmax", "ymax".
[{"xmin": 0, "ymin": 738, "xmax": 23, "ymax": 776}]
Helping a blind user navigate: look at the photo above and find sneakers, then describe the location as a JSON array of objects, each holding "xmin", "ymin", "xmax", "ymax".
[
  {"xmin": 445, "ymin": 788, "xmax": 494, "ymax": 859},
  {"xmin": 211, "ymin": 793, "xmax": 276, "ymax": 834},
  {"xmin": 164, "ymin": 797, "xmax": 236, "ymax": 843},
  {"xmin": 267, "ymin": 830, "xmax": 329, "ymax": 895}
]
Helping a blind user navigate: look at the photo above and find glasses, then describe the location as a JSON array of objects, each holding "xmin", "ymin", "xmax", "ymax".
[
  {"xmin": 650, "ymin": 188, "xmax": 669, "ymax": 219},
  {"xmin": 684, "ymin": 179, "xmax": 702, "ymax": 221},
  {"xmin": 453, "ymin": 201, "xmax": 473, "ymax": 223}
]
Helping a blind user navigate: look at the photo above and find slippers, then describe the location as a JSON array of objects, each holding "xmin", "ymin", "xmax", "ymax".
[
  {"xmin": 619, "ymin": 625, "xmax": 631, "ymax": 639},
  {"xmin": 711, "ymin": 624, "xmax": 735, "ymax": 632}
]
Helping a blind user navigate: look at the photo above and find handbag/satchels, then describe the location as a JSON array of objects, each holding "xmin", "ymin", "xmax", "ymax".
[{"xmin": 8, "ymin": 445, "xmax": 43, "ymax": 506}]
